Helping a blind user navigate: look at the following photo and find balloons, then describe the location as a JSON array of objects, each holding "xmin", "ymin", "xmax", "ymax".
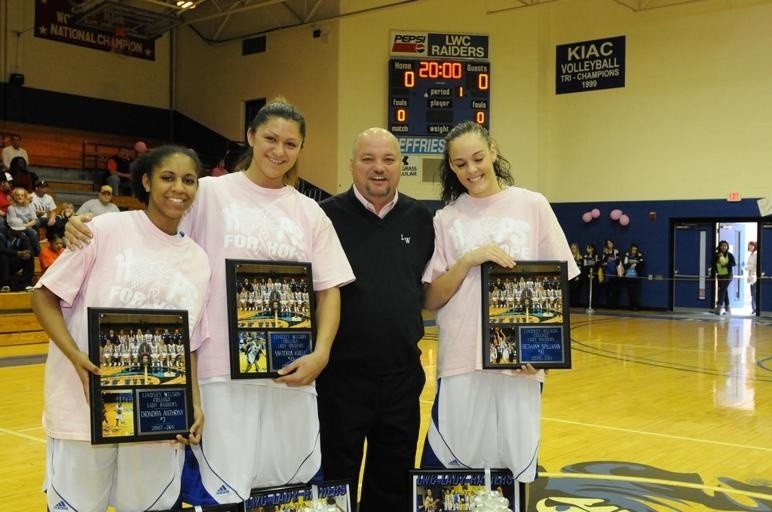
[
  {"xmin": 609, "ymin": 209, "xmax": 630, "ymax": 226},
  {"xmin": 582, "ymin": 209, "xmax": 601, "ymax": 223}
]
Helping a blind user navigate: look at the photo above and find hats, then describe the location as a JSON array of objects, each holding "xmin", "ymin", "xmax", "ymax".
[
  {"xmin": 0, "ymin": 172, "xmax": 13, "ymax": 182},
  {"xmin": 36, "ymin": 177, "xmax": 48, "ymax": 187},
  {"xmin": 101, "ymin": 185, "xmax": 112, "ymax": 194}
]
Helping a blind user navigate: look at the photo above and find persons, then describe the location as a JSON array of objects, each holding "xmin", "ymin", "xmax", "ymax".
[
  {"xmin": 64, "ymin": 98, "xmax": 354, "ymax": 512},
  {"xmin": 100, "ymin": 327, "xmax": 184, "ymax": 370},
  {"xmin": 567, "ymin": 238, "xmax": 643, "ymax": 278},
  {"xmin": 424, "ymin": 485, "xmax": 469, "ymax": 511},
  {"xmin": 239, "ymin": 333, "xmax": 269, "ymax": 373},
  {"xmin": 489, "ymin": 326, "xmax": 517, "ymax": 363},
  {"xmin": 709, "ymin": 240, "xmax": 736, "ymax": 315},
  {"xmin": 235, "ymin": 274, "xmax": 310, "ymax": 310},
  {"xmin": 745, "ymin": 241, "xmax": 758, "ymax": 313},
  {"xmin": 308, "ymin": 127, "xmax": 435, "ymax": 511},
  {"xmin": 488, "ymin": 275, "xmax": 561, "ymax": 309},
  {"xmin": 29, "ymin": 144, "xmax": 211, "ymax": 510},
  {"xmin": 0, "ymin": 135, "xmax": 237, "ymax": 293},
  {"xmin": 102, "ymin": 399, "xmax": 124, "ymax": 428},
  {"xmin": 415, "ymin": 120, "xmax": 581, "ymax": 512}
]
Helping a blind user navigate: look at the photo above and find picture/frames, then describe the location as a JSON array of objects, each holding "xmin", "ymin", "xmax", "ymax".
[
  {"xmin": 239, "ymin": 478, "xmax": 356, "ymax": 512},
  {"xmin": 407, "ymin": 468, "xmax": 525, "ymax": 512},
  {"xmin": 87, "ymin": 308, "xmax": 194, "ymax": 444},
  {"xmin": 226, "ymin": 259, "xmax": 317, "ymax": 379},
  {"xmin": 482, "ymin": 261, "xmax": 571, "ymax": 369}
]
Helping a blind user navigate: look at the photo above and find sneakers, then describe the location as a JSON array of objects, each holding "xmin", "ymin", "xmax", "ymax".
[
  {"xmin": 709, "ymin": 309, "xmax": 731, "ymax": 315},
  {"xmin": 1, "ymin": 286, "xmax": 10, "ymax": 292},
  {"xmin": 26, "ymin": 286, "xmax": 33, "ymax": 292}
]
